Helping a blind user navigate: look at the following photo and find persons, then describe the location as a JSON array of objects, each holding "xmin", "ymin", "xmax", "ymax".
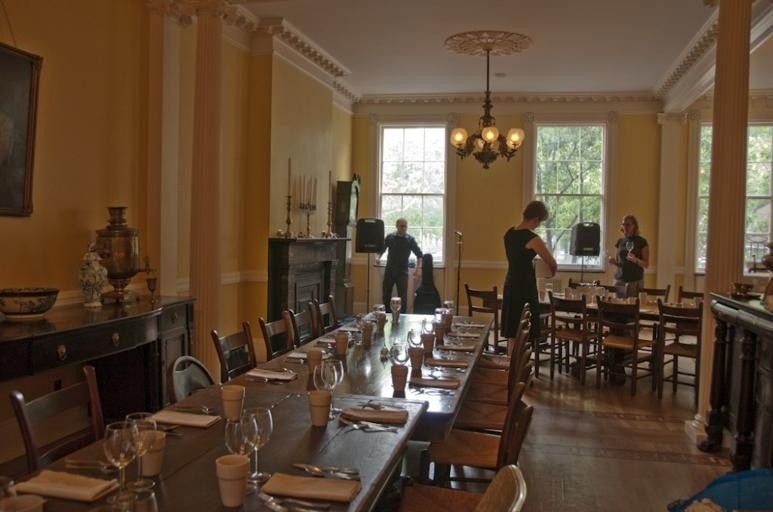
[
  {"xmin": 500, "ymin": 200, "xmax": 557, "ymax": 351},
  {"xmin": 607, "ymin": 214, "xmax": 650, "ymax": 363},
  {"xmin": 372, "ymin": 218, "xmax": 424, "ymax": 313}
]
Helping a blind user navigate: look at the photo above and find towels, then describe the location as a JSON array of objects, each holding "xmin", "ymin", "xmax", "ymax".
[
  {"xmin": 339, "ymin": 325, "xmax": 361, "ymax": 333},
  {"xmin": 16, "ymin": 469, "xmax": 121, "ymax": 502},
  {"xmin": 340, "ymin": 407, "xmax": 409, "ymax": 426},
  {"xmin": 261, "ymin": 472, "xmax": 363, "ymax": 502}
]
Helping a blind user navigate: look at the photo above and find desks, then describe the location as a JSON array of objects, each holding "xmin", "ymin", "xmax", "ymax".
[{"xmin": 0, "ymin": 295, "xmax": 196, "ymax": 439}]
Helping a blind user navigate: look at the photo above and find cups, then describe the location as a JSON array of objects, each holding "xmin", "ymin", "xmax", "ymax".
[
  {"xmin": 307, "ymin": 391, "xmax": 332, "ymax": 427},
  {"xmin": 335, "ymin": 333, "xmax": 348, "ymax": 357},
  {"xmin": 408, "ymin": 347, "xmax": 425, "ymax": 370},
  {"xmin": 393, "ymin": 338, "xmax": 410, "ymax": 362},
  {"xmin": 391, "ymin": 364, "xmax": 409, "ymax": 395},
  {"xmin": 219, "ymin": 385, "xmax": 245, "ymax": 422},
  {"xmin": 565, "ymin": 286, "xmax": 648, "ymax": 306},
  {"xmin": 307, "ymin": 350, "xmax": 323, "ymax": 372},
  {"xmin": 216, "ymin": 455, "xmax": 251, "ymax": 506},
  {"xmin": 137, "ymin": 430, "xmax": 166, "ymax": 478},
  {"xmin": 390, "ymin": 345, "xmax": 407, "ymax": 365},
  {"xmin": 314, "ymin": 304, "xmax": 456, "ymax": 352}
]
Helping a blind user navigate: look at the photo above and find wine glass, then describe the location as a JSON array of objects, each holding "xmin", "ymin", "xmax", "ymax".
[
  {"xmin": 104, "ymin": 423, "xmax": 138, "ymax": 507},
  {"xmin": 321, "ymin": 360, "xmax": 346, "ymax": 413},
  {"xmin": 123, "ymin": 413, "xmax": 156, "ymax": 492},
  {"xmin": 226, "ymin": 417, "xmax": 258, "ymax": 495},
  {"xmin": 626, "ymin": 241, "xmax": 634, "ymax": 253},
  {"xmin": 315, "ymin": 364, "xmax": 337, "ymax": 422},
  {"xmin": 242, "ymin": 408, "xmax": 274, "ymax": 483}
]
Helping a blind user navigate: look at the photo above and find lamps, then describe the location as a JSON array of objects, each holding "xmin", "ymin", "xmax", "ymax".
[{"xmin": 444, "ymin": 30, "xmax": 533, "ymax": 169}]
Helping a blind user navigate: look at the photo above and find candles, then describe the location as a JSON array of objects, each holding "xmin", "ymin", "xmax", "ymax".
[{"xmin": 286, "ymin": 158, "xmax": 332, "ymax": 205}]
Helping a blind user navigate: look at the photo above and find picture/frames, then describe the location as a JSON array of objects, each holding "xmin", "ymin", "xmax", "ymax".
[{"xmin": 0, "ymin": 42, "xmax": 43, "ymax": 217}]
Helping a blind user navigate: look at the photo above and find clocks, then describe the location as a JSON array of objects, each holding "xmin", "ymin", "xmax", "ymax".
[{"xmin": 332, "ymin": 172, "xmax": 360, "ymax": 316}]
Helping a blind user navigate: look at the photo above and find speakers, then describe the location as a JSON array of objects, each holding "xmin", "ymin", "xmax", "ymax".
[
  {"xmin": 570, "ymin": 222, "xmax": 601, "ymax": 258},
  {"xmin": 356, "ymin": 217, "xmax": 386, "ymax": 254}
]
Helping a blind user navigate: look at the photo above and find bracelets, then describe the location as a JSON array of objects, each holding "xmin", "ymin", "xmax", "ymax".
[{"xmin": 635, "ymin": 258, "xmax": 640, "ymax": 265}]
